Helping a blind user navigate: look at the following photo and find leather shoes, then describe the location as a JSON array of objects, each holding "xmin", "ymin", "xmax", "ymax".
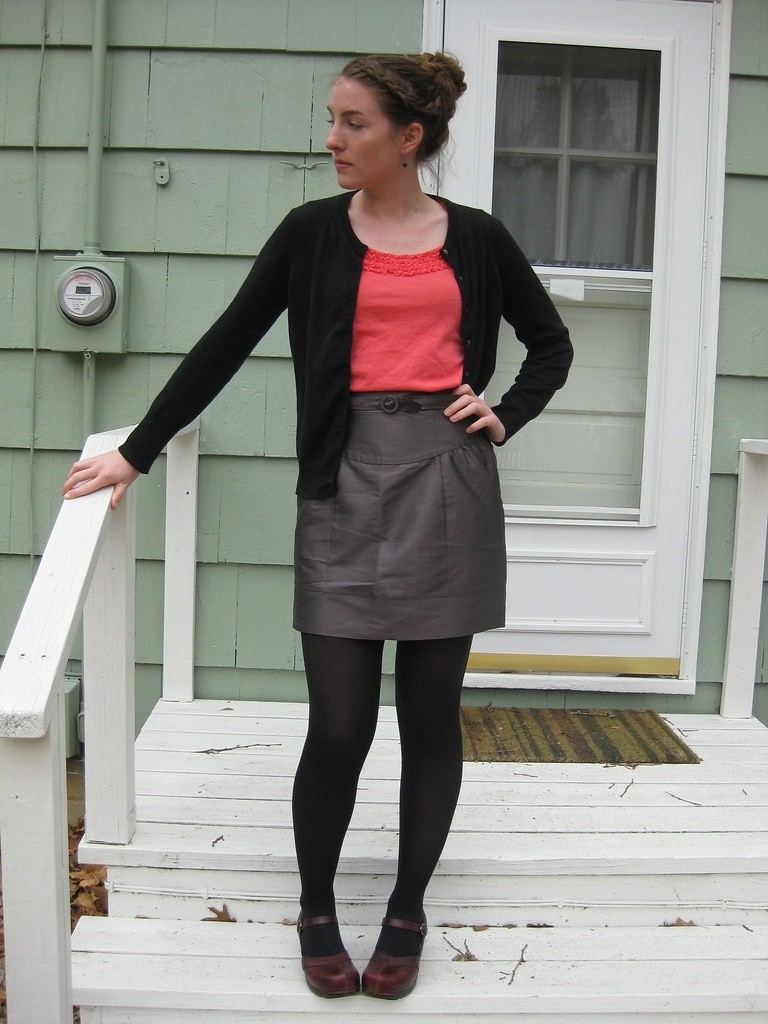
[
  {"xmin": 361, "ymin": 914, "xmax": 429, "ymax": 999},
  {"xmin": 298, "ymin": 912, "xmax": 360, "ymax": 999}
]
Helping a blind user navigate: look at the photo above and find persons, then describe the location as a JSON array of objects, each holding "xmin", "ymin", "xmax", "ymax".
[{"xmin": 60, "ymin": 50, "xmax": 575, "ymax": 1001}]
